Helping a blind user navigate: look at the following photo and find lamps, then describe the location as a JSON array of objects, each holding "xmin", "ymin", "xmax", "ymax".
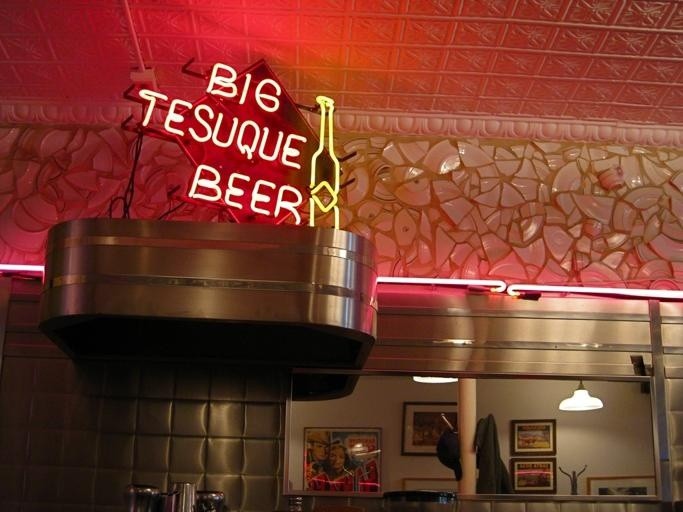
[{"xmin": 558, "ymin": 380, "xmax": 604, "ymax": 411}]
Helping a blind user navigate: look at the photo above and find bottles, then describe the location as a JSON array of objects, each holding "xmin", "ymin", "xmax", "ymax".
[
  {"xmin": 122, "ymin": 482, "xmax": 226, "ymax": 512},
  {"xmin": 287, "ymin": 497, "xmax": 304, "ymax": 511},
  {"xmin": 310, "ymin": 94, "xmax": 343, "ymax": 231}
]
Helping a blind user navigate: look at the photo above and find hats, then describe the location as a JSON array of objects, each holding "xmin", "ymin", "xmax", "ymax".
[{"xmin": 436, "ymin": 427, "xmax": 462, "ymax": 481}]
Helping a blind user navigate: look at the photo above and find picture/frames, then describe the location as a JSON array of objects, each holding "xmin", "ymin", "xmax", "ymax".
[
  {"xmin": 401, "ymin": 402, "xmax": 457, "ymax": 456},
  {"xmin": 509, "ymin": 418, "xmax": 557, "ymax": 494},
  {"xmin": 302, "ymin": 426, "xmax": 383, "ymax": 492},
  {"xmin": 586, "ymin": 476, "xmax": 658, "ymax": 495}
]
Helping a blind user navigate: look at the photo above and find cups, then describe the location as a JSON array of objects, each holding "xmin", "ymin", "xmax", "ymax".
[{"xmin": 597, "ymin": 165, "xmax": 622, "ymax": 193}]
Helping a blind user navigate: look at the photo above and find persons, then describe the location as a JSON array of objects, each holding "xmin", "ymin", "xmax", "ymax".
[
  {"xmin": 307, "ymin": 430, "xmax": 329, "ymax": 476},
  {"xmin": 306, "ymin": 440, "xmax": 358, "ymax": 491}
]
